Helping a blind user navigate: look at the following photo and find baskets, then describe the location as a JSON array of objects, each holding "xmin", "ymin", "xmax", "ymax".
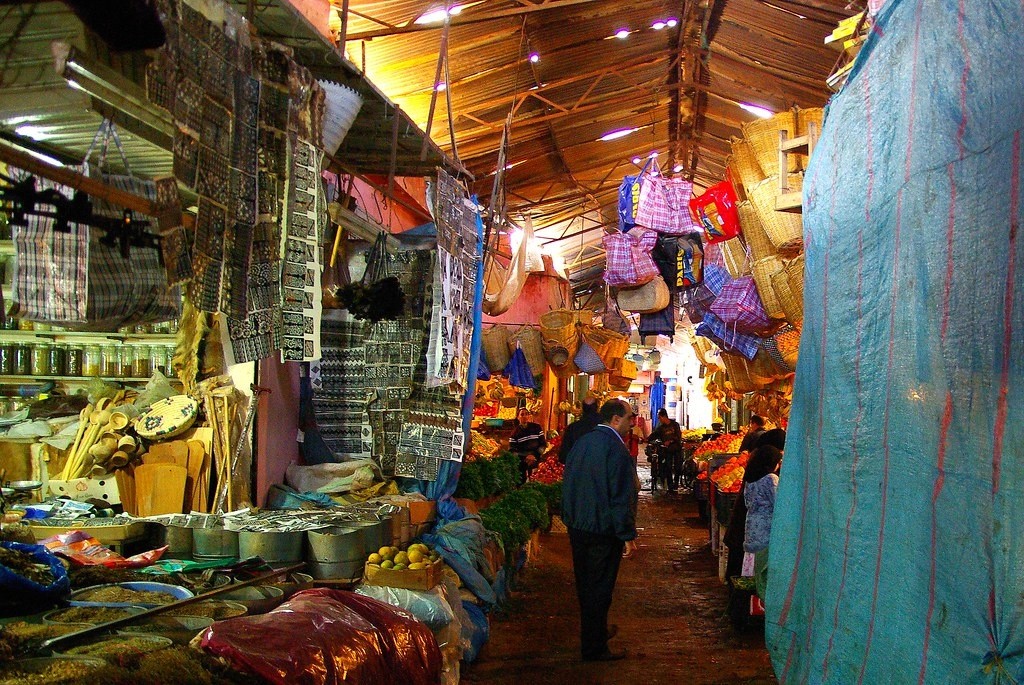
[
  {"xmin": 480, "ymin": 322, "xmax": 511, "ymax": 372},
  {"xmin": 692, "ymin": 107, "xmax": 826, "ymax": 393},
  {"xmin": 506, "ymin": 323, "xmax": 545, "ymax": 377},
  {"xmin": 538, "ymin": 308, "xmax": 638, "ymax": 389}
]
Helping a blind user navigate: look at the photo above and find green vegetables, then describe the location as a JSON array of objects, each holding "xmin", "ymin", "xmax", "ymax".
[{"xmin": 463, "ymin": 451, "xmax": 563, "ymax": 546}]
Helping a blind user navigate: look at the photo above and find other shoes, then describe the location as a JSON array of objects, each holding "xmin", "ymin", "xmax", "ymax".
[
  {"xmin": 606, "ymin": 623, "xmax": 618, "ymax": 641},
  {"xmin": 667, "ymin": 489, "xmax": 674, "ymax": 494},
  {"xmin": 584, "ymin": 647, "xmax": 627, "ymax": 660}
]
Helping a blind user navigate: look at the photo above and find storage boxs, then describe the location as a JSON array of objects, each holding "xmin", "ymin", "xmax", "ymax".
[
  {"xmin": 364, "ymin": 555, "xmax": 446, "ymax": 591},
  {"xmin": 48, "ymin": 468, "xmax": 122, "ymax": 508},
  {"xmin": 693, "ymin": 477, "xmax": 741, "ymax": 527},
  {"xmin": 27, "ymin": 520, "xmax": 147, "ymax": 540},
  {"xmin": 728, "ymin": 576, "xmax": 756, "ymax": 619},
  {"xmin": 549, "ymin": 515, "xmax": 567, "ymax": 533},
  {"xmin": 388, "ymin": 499, "xmax": 437, "ymax": 523}
]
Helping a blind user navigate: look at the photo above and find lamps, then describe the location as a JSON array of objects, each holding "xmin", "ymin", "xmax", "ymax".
[{"xmin": 326, "ymin": 201, "xmax": 401, "ymax": 256}]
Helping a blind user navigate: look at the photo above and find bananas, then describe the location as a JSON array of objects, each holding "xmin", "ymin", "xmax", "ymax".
[
  {"xmin": 560, "ymin": 402, "xmax": 570, "ymax": 411},
  {"xmin": 708, "ymin": 383, "xmax": 792, "ymax": 418},
  {"xmin": 587, "ymin": 380, "xmax": 611, "ymax": 400},
  {"xmin": 526, "ymin": 399, "xmax": 543, "ymax": 413},
  {"xmin": 489, "ymin": 388, "xmax": 505, "ymax": 398}
]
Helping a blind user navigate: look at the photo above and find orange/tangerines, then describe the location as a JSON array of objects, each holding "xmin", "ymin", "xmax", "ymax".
[{"xmin": 368, "ymin": 544, "xmax": 435, "ymax": 570}]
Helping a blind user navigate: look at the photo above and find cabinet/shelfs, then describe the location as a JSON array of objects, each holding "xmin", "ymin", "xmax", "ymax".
[{"xmin": 0, "ymin": 282, "xmax": 181, "ymax": 382}]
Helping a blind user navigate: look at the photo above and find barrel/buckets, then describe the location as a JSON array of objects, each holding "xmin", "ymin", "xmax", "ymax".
[{"xmin": 0, "ymin": 505, "xmax": 410, "ymax": 685}]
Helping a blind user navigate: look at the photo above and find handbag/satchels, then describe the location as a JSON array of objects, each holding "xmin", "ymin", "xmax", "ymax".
[
  {"xmin": 601, "ymin": 153, "xmax": 771, "ymax": 361},
  {"xmin": 6, "ymin": 118, "xmax": 183, "ymax": 331}
]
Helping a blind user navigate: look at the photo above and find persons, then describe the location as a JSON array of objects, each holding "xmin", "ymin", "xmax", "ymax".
[
  {"xmin": 741, "ymin": 416, "xmax": 769, "ymax": 452},
  {"xmin": 558, "ymin": 396, "xmax": 600, "ymax": 465},
  {"xmin": 643, "ymin": 408, "xmax": 683, "ymax": 495},
  {"xmin": 508, "ymin": 406, "xmax": 546, "ymax": 486},
  {"xmin": 722, "ymin": 429, "xmax": 787, "ymax": 592},
  {"xmin": 742, "ymin": 447, "xmax": 782, "ymax": 615},
  {"xmin": 560, "ymin": 399, "xmax": 643, "ymax": 661}
]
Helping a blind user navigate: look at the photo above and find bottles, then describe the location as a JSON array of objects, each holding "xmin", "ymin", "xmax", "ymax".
[{"xmin": 90, "ymin": 509, "xmax": 114, "ymax": 517}]
